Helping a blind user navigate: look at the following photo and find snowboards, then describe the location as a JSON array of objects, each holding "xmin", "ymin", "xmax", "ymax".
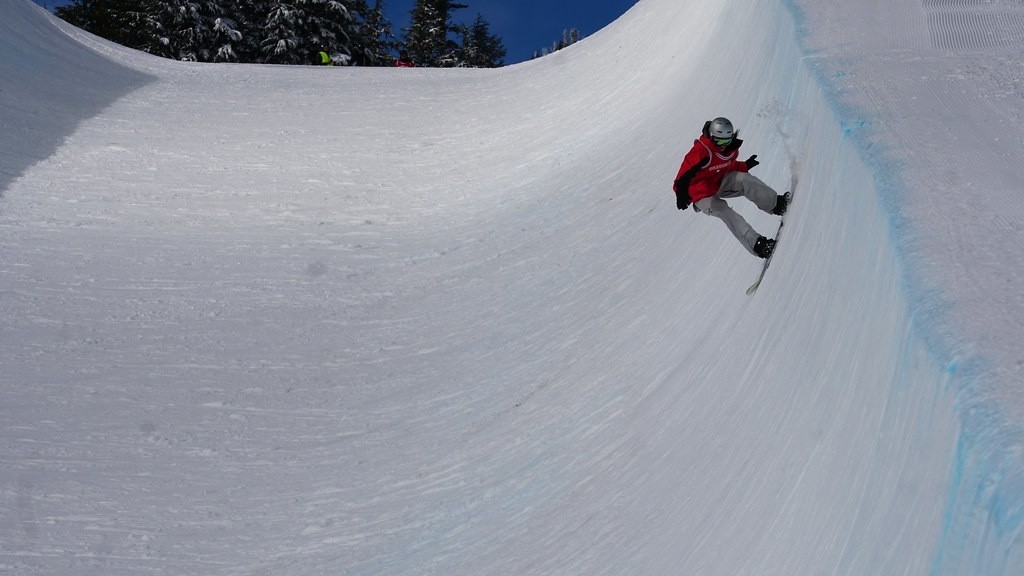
[{"xmin": 744, "ymin": 182, "xmax": 788, "ymax": 297}]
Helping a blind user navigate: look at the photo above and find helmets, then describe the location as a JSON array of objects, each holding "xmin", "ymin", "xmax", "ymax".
[{"xmin": 709, "ymin": 117, "xmax": 733, "ymax": 138}]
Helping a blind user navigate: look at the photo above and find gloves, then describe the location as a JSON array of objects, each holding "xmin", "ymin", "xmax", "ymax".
[
  {"xmin": 676, "ymin": 188, "xmax": 692, "ymax": 210},
  {"xmin": 745, "ymin": 155, "xmax": 759, "ymax": 170}
]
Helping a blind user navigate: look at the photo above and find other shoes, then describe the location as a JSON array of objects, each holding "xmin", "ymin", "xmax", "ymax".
[
  {"xmin": 753, "ymin": 236, "xmax": 775, "ymax": 259},
  {"xmin": 771, "ymin": 191, "xmax": 790, "ymax": 215}
]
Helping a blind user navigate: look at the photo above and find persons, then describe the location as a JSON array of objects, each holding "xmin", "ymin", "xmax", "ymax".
[
  {"xmin": 673, "ymin": 117, "xmax": 791, "ymax": 258},
  {"xmin": 394, "ymin": 50, "xmax": 413, "ymax": 67},
  {"xmin": 316, "ymin": 42, "xmax": 333, "ymax": 66}
]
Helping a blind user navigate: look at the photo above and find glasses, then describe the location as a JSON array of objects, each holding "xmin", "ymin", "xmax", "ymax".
[{"xmin": 711, "ymin": 136, "xmax": 733, "ymax": 146}]
